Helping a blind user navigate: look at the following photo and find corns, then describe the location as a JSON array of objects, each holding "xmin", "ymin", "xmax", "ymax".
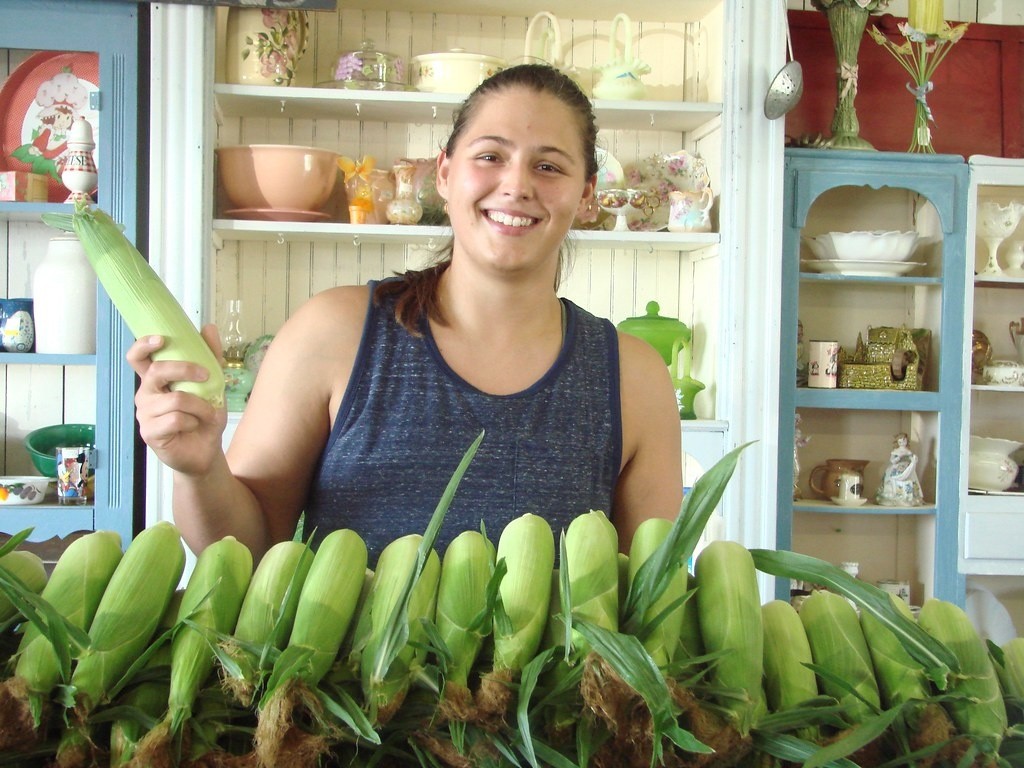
[
  {"xmin": 0, "ymin": 511, "xmax": 1024, "ymax": 768},
  {"xmin": 72, "ymin": 197, "xmax": 224, "ymax": 409}
]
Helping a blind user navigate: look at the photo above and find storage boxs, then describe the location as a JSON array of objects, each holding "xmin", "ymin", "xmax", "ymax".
[{"xmin": 0, "ymin": 170, "xmax": 50, "ymax": 203}]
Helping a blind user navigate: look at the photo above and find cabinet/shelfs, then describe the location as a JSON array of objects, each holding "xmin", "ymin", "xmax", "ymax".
[
  {"xmin": 146, "ymin": 2, "xmax": 786, "ymax": 610},
  {"xmin": 778, "ymin": 142, "xmax": 969, "ymax": 603},
  {"xmin": 956, "ymin": 154, "xmax": 1024, "ymax": 578},
  {"xmin": 0, "ymin": 1, "xmax": 136, "ymax": 555}
]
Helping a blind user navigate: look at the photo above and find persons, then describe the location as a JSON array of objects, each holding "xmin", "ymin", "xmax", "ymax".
[
  {"xmin": 793, "ymin": 412, "xmax": 811, "ymax": 502},
  {"xmin": 873, "ymin": 432, "xmax": 925, "ymax": 507},
  {"xmin": 124, "ymin": 57, "xmax": 684, "ymax": 668}
]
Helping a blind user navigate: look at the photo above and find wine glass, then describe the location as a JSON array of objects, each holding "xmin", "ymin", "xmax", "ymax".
[
  {"xmin": 975, "ymin": 201, "xmax": 1024, "ymax": 276},
  {"xmin": 598, "ymin": 188, "xmax": 646, "ymax": 232}
]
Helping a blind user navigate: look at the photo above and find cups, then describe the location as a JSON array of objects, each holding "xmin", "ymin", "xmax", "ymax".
[
  {"xmin": 0, "ymin": 298, "xmax": 35, "ymax": 353},
  {"xmin": 55, "ymin": 444, "xmax": 90, "ymax": 504},
  {"xmin": 877, "ymin": 579, "xmax": 911, "ymax": 607},
  {"xmin": 838, "ymin": 474, "xmax": 860, "ymax": 498},
  {"xmin": 668, "ymin": 188, "xmax": 713, "ymax": 233},
  {"xmin": 809, "ymin": 340, "xmax": 838, "ymax": 388}
]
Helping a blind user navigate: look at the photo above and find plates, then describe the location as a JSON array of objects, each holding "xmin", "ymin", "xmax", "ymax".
[
  {"xmin": 830, "ymin": 496, "xmax": 867, "ymax": 505},
  {"xmin": 800, "ymin": 257, "xmax": 927, "ymax": 275},
  {"xmin": 227, "ymin": 208, "xmax": 329, "ymax": 222},
  {"xmin": 570, "ymin": 147, "xmax": 711, "ymax": 232}
]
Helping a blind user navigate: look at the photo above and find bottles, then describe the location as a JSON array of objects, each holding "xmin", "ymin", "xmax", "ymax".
[
  {"xmin": 1006, "ymin": 240, "xmax": 1024, "ymax": 268},
  {"xmin": 969, "ymin": 435, "xmax": 1024, "ymax": 492},
  {"xmin": 386, "ymin": 165, "xmax": 424, "ymax": 226}
]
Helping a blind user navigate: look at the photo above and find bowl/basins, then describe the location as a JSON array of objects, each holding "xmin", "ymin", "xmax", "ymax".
[
  {"xmin": 23, "ymin": 425, "xmax": 95, "ymax": 499},
  {"xmin": 215, "ymin": 144, "xmax": 342, "ymax": 211},
  {"xmin": 616, "ymin": 301, "xmax": 691, "ymax": 365},
  {"xmin": 0, "ymin": 475, "xmax": 48, "ymax": 504},
  {"xmin": 801, "ymin": 230, "xmax": 921, "ymax": 262},
  {"xmin": 413, "ymin": 46, "xmax": 508, "ymax": 95},
  {"xmin": 983, "ymin": 366, "xmax": 1023, "ymax": 386}
]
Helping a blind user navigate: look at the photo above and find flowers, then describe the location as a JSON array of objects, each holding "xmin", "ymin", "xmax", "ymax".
[
  {"xmin": 808, "ymin": 0, "xmax": 890, "ymax": 19},
  {"xmin": 867, "ymin": 0, "xmax": 969, "ymax": 155}
]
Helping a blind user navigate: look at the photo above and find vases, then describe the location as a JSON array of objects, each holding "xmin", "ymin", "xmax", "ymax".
[{"xmin": 820, "ymin": 7, "xmax": 881, "ymax": 153}]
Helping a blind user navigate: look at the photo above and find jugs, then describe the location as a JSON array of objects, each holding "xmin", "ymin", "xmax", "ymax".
[{"xmin": 809, "ymin": 459, "xmax": 870, "ymax": 498}]
